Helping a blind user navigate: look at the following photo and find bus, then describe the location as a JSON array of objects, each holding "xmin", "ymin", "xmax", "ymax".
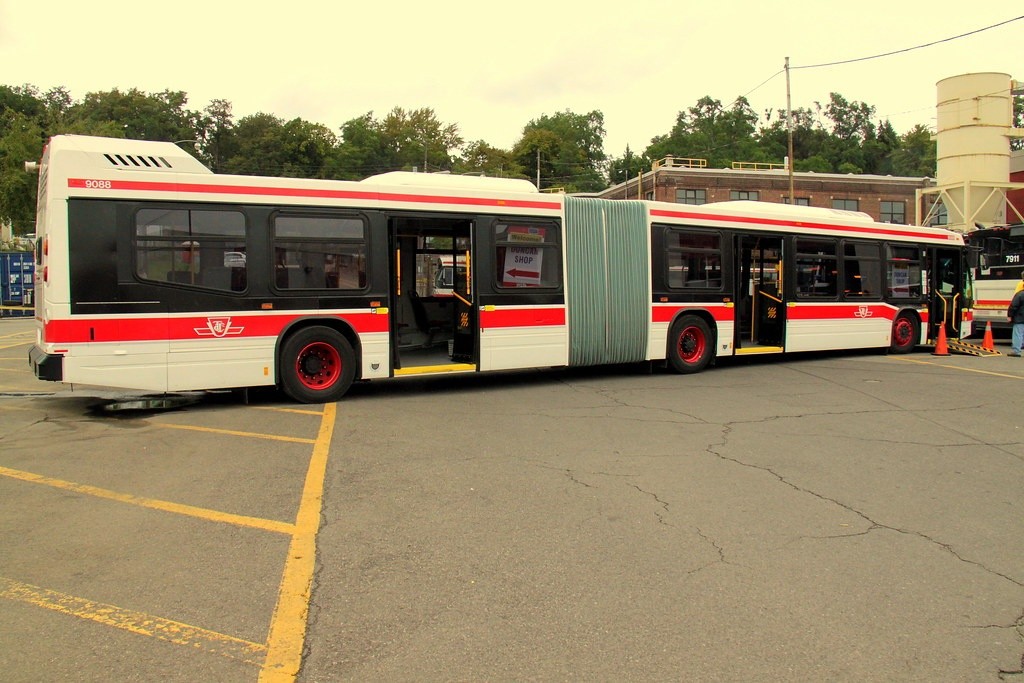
[
  {"xmin": 24, "ymin": 134, "xmax": 969, "ymax": 412},
  {"xmin": 964, "ymin": 220, "xmax": 1023, "ymax": 336}
]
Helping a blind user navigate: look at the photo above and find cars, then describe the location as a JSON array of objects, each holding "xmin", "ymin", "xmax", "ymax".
[{"xmin": 19, "ymin": 233, "xmax": 35, "ymax": 245}]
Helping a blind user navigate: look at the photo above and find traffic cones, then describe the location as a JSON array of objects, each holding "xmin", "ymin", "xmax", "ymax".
[
  {"xmin": 931, "ymin": 321, "xmax": 951, "ymax": 356},
  {"xmin": 982, "ymin": 321, "xmax": 994, "ymax": 349}
]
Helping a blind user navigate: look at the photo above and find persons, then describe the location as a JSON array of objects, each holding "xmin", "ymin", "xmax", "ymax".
[{"xmin": 1007, "ymin": 290, "xmax": 1024, "ymax": 357}]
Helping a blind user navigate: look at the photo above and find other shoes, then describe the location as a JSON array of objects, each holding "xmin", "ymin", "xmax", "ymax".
[{"xmin": 1007, "ymin": 353, "xmax": 1021, "ymax": 357}]
